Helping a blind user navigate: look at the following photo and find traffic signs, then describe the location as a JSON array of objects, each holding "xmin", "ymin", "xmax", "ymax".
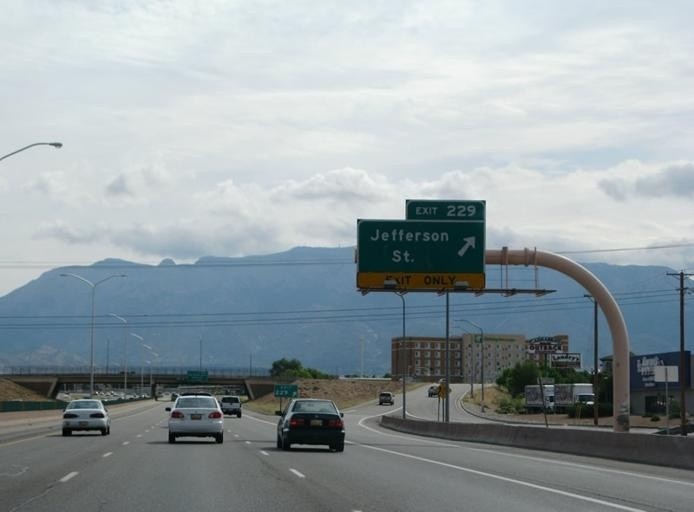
[{"xmin": 353, "ymin": 200, "xmax": 488, "ymax": 298}]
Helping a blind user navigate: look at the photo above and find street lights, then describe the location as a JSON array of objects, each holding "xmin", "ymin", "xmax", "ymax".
[{"xmin": 59, "ymin": 271, "xmax": 164, "ymax": 404}]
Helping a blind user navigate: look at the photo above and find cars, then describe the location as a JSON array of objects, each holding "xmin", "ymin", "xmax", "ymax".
[
  {"xmin": 165, "ymin": 392, "xmax": 224, "ymax": 444},
  {"xmin": 379, "ymin": 391, "xmax": 396, "ymax": 405},
  {"xmin": 218, "ymin": 395, "xmax": 242, "ymax": 418},
  {"xmin": 62, "ymin": 399, "xmax": 111, "ymax": 436},
  {"xmin": 428, "ymin": 386, "xmax": 441, "ymax": 398},
  {"xmin": 276, "ymin": 398, "xmax": 345, "ymax": 452},
  {"xmin": 439, "ymin": 378, "xmax": 447, "ymax": 384}
]
